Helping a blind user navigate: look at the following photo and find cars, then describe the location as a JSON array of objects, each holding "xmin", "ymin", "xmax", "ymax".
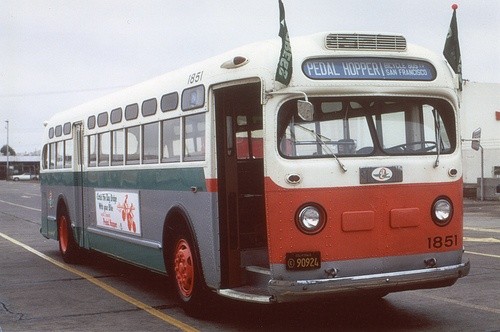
[{"xmin": 10, "ymin": 172, "xmax": 39, "ymax": 182}]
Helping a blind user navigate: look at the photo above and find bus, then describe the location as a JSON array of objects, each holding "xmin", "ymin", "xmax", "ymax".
[{"xmin": 39, "ymin": 31, "xmax": 470, "ymax": 316}]
